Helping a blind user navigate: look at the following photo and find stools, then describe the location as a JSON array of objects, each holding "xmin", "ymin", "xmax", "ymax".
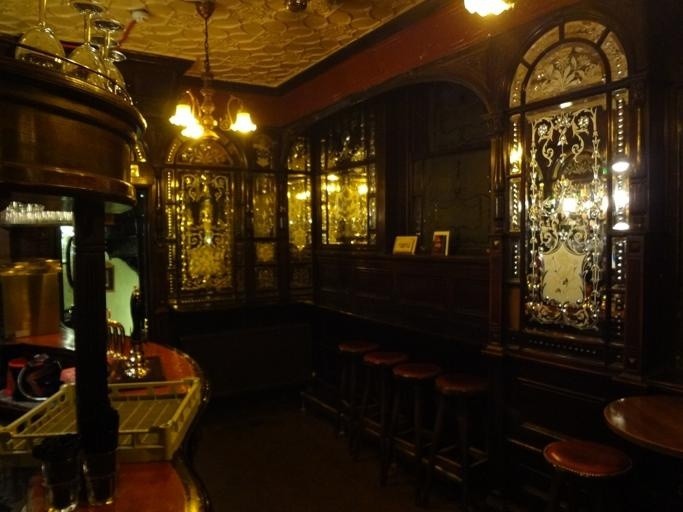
[
  {"xmin": 542, "ymin": 441, "xmax": 634, "ymax": 509},
  {"xmin": 331, "ymin": 339, "xmax": 497, "ymax": 510}
]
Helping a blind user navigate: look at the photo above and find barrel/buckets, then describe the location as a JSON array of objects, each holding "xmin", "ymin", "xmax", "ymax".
[{"xmin": 1, "ymin": 256, "xmax": 68, "ymax": 340}]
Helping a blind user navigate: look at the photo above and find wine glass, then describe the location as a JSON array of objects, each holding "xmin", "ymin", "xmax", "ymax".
[{"xmin": 16, "ymin": 0, "xmax": 128, "ymax": 96}]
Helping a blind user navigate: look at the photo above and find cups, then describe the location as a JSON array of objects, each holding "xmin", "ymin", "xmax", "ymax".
[{"xmin": 40, "ymin": 462, "xmax": 118, "ymax": 511}]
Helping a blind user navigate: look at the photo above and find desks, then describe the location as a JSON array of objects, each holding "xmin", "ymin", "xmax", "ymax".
[{"xmin": 603, "ymin": 393, "xmax": 683, "ymax": 510}]
